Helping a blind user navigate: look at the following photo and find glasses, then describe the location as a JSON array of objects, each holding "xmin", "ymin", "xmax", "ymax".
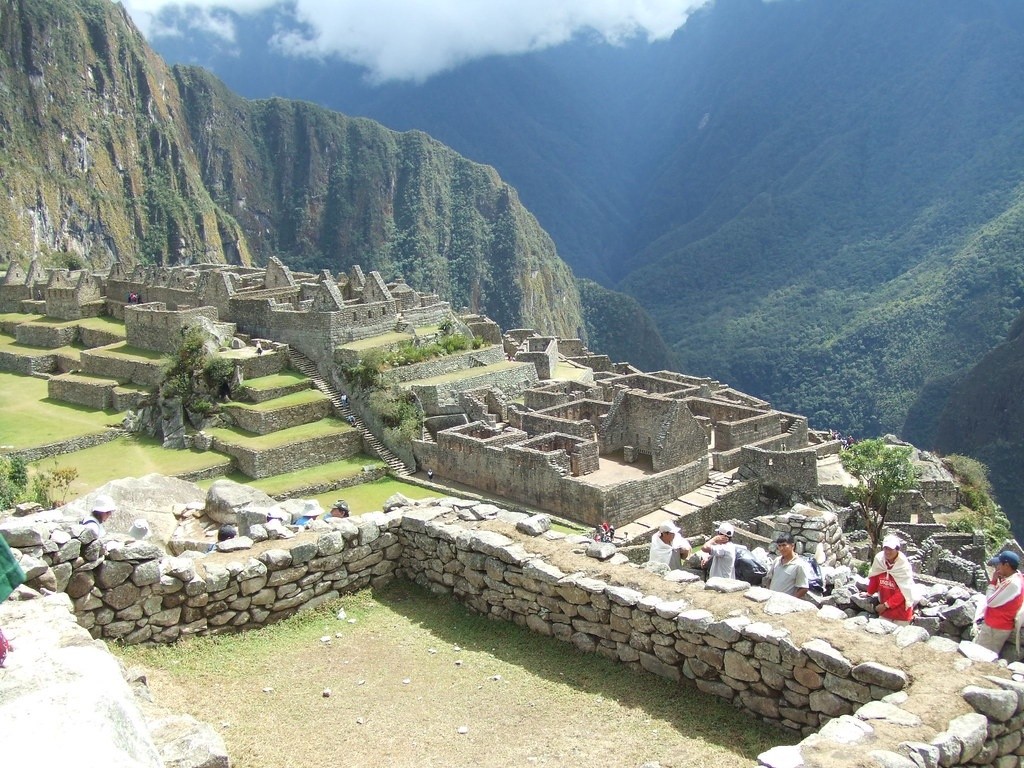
[{"xmin": 106, "ymin": 511, "xmax": 111, "ymax": 514}]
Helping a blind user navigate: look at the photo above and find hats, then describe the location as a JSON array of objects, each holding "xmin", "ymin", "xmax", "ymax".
[
  {"xmin": 715, "ymin": 523, "xmax": 734, "ymax": 537},
  {"xmin": 129, "ymin": 519, "xmax": 152, "ymax": 540},
  {"xmin": 660, "ymin": 520, "xmax": 681, "ymax": 534},
  {"xmin": 987, "ymin": 551, "xmax": 1020, "ymax": 567},
  {"xmin": 329, "ymin": 500, "xmax": 349, "ymax": 511},
  {"xmin": 92, "ymin": 496, "xmax": 117, "ymax": 512},
  {"xmin": 882, "ymin": 535, "xmax": 900, "ymax": 549},
  {"xmin": 299, "ymin": 500, "xmax": 325, "ymax": 516}
]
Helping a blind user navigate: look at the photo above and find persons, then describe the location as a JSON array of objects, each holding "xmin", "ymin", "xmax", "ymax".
[
  {"xmin": 649, "ymin": 520, "xmax": 692, "ymax": 570},
  {"xmin": 859, "ymin": 535, "xmax": 914, "ymax": 627},
  {"xmin": 37, "ymin": 290, "xmax": 42, "ymax": 300},
  {"xmin": 428, "ymin": 469, "xmax": 433, "ymax": 482},
  {"xmin": 595, "ymin": 523, "xmax": 615, "ymax": 543},
  {"xmin": 83, "ymin": 494, "xmax": 325, "ymax": 554},
  {"xmin": 341, "ymin": 394, "xmax": 356, "ymax": 426},
  {"xmin": 766, "ymin": 534, "xmax": 809, "ymax": 598},
  {"xmin": 128, "ymin": 292, "xmax": 143, "ymax": 304},
  {"xmin": 257, "ymin": 341, "xmax": 262, "ymax": 355},
  {"xmin": 972, "ymin": 551, "xmax": 1024, "ymax": 655},
  {"xmin": 326, "ymin": 499, "xmax": 350, "ymax": 518},
  {"xmin": 781, "ymin": 429, "xmax": 854, "ymax": 451},
  {"xmin": 701, "ymin": 523, "xmax": 735, "ymax": 579}
]
